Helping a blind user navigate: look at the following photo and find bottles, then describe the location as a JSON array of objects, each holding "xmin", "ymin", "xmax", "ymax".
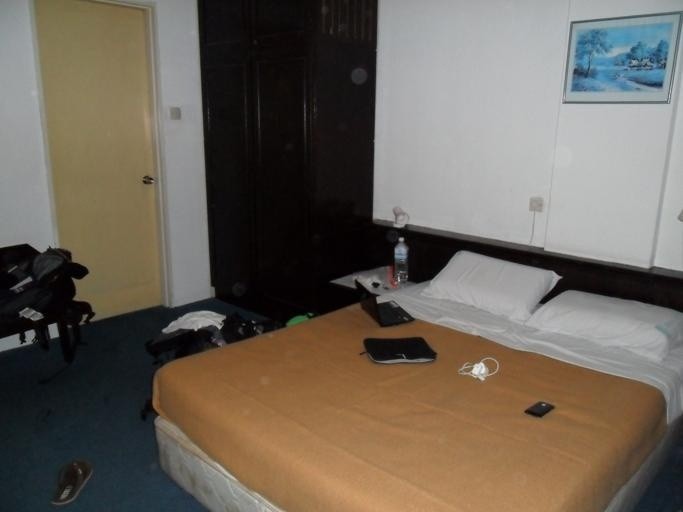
[{"xmin": 392, "ymin": 236, "xmax": 410, "ymax": 283}]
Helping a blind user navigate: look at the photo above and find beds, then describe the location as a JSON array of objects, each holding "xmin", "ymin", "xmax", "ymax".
[{"xmin": 150, "ymin": 281, "xmax": 682, "ymax": 512}]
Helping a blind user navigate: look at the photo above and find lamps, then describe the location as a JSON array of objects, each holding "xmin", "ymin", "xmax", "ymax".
[{"xmin": 391, "ymin": 206, "xmax": 409, "ymax": 229}]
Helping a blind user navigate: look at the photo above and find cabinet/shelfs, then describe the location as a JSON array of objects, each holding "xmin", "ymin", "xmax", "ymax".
[{"xmin": 195, "ymin": 0, "xmax": 377, "ymax": 324}]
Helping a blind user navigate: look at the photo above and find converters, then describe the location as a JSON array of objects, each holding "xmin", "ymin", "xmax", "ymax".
[{"xmin": 472, "ymin": 362, "xmax": 490, "ymax": 378}]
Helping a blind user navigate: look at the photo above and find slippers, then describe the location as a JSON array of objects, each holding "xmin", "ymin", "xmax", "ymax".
[{"xmin": 49, "ymin": 456, "xmax": 94, "ymax": 506}]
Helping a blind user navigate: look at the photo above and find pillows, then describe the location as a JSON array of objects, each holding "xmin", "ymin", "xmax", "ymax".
[
  {"xmin": 524, "ymin": 288, "xmax": 683, "ymax": 363},
  {"xmin": 418, "ymin": 248, "xmax": 566, "ymax": 325}
]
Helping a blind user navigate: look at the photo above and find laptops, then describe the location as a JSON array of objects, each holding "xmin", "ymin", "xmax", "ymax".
[{"xmin": 354, "ymin": 278, "xmax": 415, "ymax": 329}]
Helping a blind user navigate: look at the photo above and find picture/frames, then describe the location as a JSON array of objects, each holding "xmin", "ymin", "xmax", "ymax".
[{"xmin": 559, "ymin": 8, "xmax": 683, "ymax": 105}]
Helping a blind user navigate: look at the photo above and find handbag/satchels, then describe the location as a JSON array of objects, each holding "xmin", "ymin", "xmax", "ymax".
[{"xmin": 359, "ymin": 336, "xmax": 438, "ymax": 366}]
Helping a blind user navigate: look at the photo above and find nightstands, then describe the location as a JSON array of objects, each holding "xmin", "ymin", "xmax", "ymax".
[{"xmin": 325, "ymin": 266, "xmax": 417, "ymax": 313}]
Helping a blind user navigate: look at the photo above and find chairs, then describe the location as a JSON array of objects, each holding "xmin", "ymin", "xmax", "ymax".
[{"xmin": 0, "ymin": 243, "xmax": 94, "ymax": 364}]
